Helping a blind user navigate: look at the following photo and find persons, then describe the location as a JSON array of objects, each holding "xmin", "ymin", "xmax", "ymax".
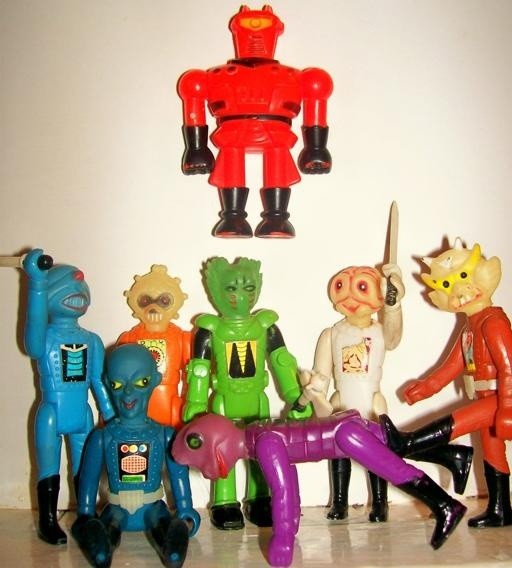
[
  {"xmin": 24, "ymin": 238, "xmax": 509, "ymax": 568},
  {"xmin": 176, "ymin": 0, "xmax": 333, "ymax": 243},
  {"xmin": 0, "ymin": 199, "xmax": 510, "ymax": 567}
]
